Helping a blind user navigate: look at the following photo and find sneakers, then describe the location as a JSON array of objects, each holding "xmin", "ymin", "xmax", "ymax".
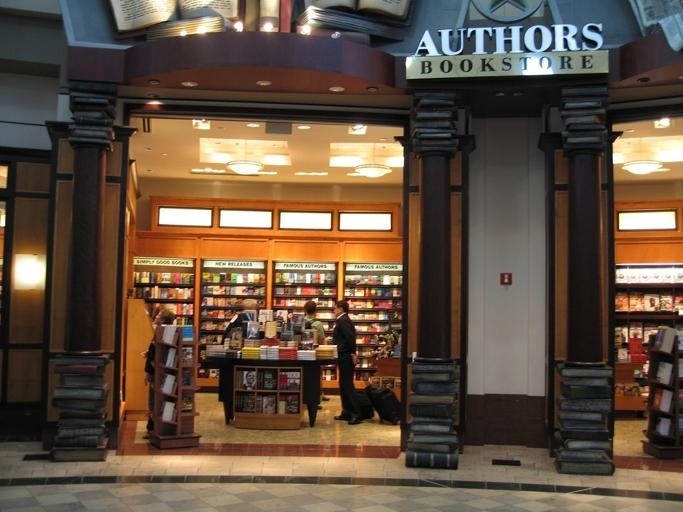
[{"xmin": 316, "ymin": 397, "xmax": 330, "ymax": 409}]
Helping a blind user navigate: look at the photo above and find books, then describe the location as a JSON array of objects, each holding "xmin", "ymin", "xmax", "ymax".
[
  {"xmin": 400, "ymin": 355, "xmax": 461, "ymax": 471},
  {"xmin": 553, "ymin": 357, "xmax": 618, "ymax": 478},
  {"xmin": 613, "ymin": 287, "xmax": 682, "ymax": 440},
  {"xmin": 44, "ymin": 345, "xmax": 116, "ymax": 465}
]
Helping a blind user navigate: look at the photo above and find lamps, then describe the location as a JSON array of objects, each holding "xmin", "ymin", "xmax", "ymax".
[
  {"xmin": 621, "ymin": 139, "xmax": 666, "ymax": 178},
  {"xmin": 354, "ymin": 142, "xmax": 393, "ymax": 179},
  {"xmin": 228, "ymin": 140, "xmax": 258, "ymax": 173}
]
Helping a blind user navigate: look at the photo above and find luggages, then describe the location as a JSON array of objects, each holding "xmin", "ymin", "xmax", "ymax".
[{"xmin": 365, "ymin": 384, "xmax": 401, "ymax": 422}]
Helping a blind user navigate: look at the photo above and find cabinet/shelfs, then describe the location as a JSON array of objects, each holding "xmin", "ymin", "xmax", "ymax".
[
  {"xmin": 273, "ymin": 258, "xmax": 399, "ymax": 389},
  {"xmin": 132, "ymin": 260, "xmax": 270, "ymax": 389},
  {"xmin": 235, "ymin": 367, "xmax": 305, "ymax": 430},
  {"xmin": 150, "ymin": 326, "xmax": 202, "ymax": 448},
  {"xmin": 613, "ymin": 263, "xmax": 683, "ymax": 460}
]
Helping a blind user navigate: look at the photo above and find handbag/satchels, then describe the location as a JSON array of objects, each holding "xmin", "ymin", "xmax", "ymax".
[{"xmin": 357, "ymin": 390, "xmax": 373, "ymax": 419}]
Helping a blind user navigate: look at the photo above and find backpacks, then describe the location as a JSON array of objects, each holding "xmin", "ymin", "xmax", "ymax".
[{"xmin": 304, "ymin": 318, "xmax": 319, "ymax": 329}]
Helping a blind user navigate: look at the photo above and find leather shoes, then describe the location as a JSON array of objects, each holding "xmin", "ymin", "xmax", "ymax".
[{"xmin": 333, "ymin": 414, "xmax": 360, "ymax": 424}]
